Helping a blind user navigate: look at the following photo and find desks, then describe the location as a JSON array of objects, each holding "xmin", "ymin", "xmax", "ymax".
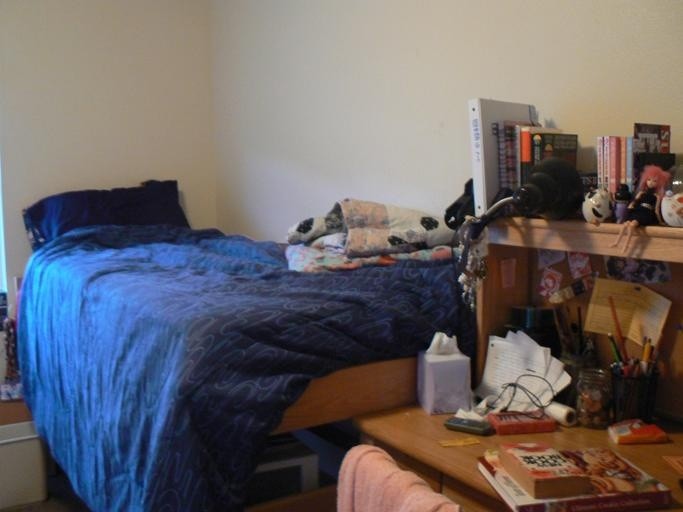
[{"xmin": 349, "ymin": 215, "xmax": 683, "ymax": 512}]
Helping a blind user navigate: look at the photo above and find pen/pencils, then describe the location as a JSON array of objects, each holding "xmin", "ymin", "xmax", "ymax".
[{"xmin": 606, "ymin": 332, "xmax": 656, "ymax": 421}]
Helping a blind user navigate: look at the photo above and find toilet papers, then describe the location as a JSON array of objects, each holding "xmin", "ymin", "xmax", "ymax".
[{"xmin": 543, "ymin": 401, "xmax": 576, "ymax": 427}]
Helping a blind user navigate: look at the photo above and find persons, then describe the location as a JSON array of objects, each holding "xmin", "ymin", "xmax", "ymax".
[{"xmin": 610, "ymin": 165, "xmax": 671, "ymax": 255}]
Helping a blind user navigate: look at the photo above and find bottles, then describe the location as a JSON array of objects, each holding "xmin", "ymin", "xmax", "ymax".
[
  {"xmin": 0, "ymin": 291, "xmax": 21, "ymax": 385},
  {"xmin": 576, "ymin": 369, "xmax": 610, "ymax": 430}
]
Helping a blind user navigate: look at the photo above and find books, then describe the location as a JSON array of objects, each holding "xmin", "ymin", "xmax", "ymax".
[
  {"xmin": 597, "ymin": 122, "xmax": 670, "ymax": 209},
  {"xmin": 477, "ymin": 446, "xmax": 669, "ymax": 512},
  {"xmin": 469, "ymin": 97, "xmax": 577, "ymax": 218}
]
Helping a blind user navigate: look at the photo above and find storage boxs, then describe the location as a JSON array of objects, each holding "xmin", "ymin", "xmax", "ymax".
[{"xmin": 0, "ymin": 400, "xmax": 47, "ymax": 508}]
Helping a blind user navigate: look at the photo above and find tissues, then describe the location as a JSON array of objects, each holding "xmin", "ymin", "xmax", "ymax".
[{"xmin": 416, "ymin": 332, "xmax": 472, "ymax": 415}]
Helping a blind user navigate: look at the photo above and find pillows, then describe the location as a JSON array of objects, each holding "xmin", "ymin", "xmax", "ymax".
[{"xmin": 21, "ymin": 181, "xmax": 189, "ymax": 252}]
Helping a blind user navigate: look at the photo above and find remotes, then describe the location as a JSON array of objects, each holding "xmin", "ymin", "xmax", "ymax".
[{"xmin": 447, "ymin": 416, "xmax": 492, "ymax": 435}]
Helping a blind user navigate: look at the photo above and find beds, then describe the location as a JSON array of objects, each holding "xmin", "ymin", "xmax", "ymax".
[{"xmin": 18, "ymin": 224, "xmax": 478, "ymax": 511}]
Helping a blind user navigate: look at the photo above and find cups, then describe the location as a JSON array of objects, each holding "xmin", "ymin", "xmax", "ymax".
[{"xmin": 611, "ymin": 368, "xmax": 658, "ymax": 422}]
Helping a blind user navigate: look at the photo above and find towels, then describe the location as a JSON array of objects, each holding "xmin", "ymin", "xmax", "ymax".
[{"xmin": 336, "ymin": 443, "xmax": 464, "ymax": 508}]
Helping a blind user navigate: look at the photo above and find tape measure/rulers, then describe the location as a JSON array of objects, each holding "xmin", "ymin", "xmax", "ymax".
[{"xmin": 663, "ymin": 455, "xmax": 682, "ymax": 477}]
